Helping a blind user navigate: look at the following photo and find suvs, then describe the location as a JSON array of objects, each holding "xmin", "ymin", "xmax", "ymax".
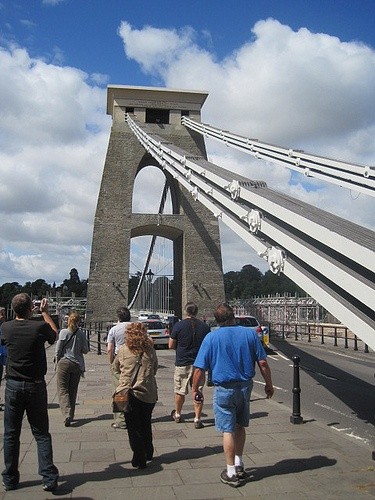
[{"xmin": 206, "ymin": 315, "xmax": 263, "ymax": 349}]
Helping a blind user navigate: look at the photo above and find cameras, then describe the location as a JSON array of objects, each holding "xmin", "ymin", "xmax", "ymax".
[{"xmin": 34, "ymin": 300, "xmax": 41, "ymax": 307}]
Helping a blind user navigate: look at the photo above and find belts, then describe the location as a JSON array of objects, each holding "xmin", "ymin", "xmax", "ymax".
[{"xmin": 7, "ymin": 376, "xmax": 44, "ymax": 382}]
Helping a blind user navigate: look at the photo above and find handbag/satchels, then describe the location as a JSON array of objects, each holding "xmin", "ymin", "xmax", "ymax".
[{"xmin": 105, "ymin": 397, "xmax": 133, "ymax": 412}]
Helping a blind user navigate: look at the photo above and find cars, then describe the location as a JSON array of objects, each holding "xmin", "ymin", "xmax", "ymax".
[
  {"xmin": 147, "ymin": 315, "xmax": 160, "ymax": 319},
  {"xmin": 139, "ymin": 320, "xmax": 169, "ymax": 349},
  {"xmin": 138, "ymin": 313, "xmax": 148, "ymax": 320}
]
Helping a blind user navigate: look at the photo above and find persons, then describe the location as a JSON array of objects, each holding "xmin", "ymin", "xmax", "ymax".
[
  {"xmin": 0, "ymin": 307, "xmax": 7, "ymax": 411},
  {"xmin": 106, "ymin": 307, "xmax": 133, "ymax": 429},
  {"xmin": 53, "ymin": 313, "xmax": 89, "ymax": 427},
  {"xmin": 110, "ymin": 321, "xmax": 158, "ymax": 468},
  {"xmin": 1, "ymin": 293, "xmax": 59, "ymax": 490},
  {"xmin": 168, "ymin": 302, "xmax": 211, "ymax": 429},
  {"xmin": 191, "ymin": 303, "xmax": 274, "ymax": 487}
]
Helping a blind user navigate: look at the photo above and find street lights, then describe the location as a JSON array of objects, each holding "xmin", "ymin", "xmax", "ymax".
[{"xmin": 145, "ymin": 269, "xmax": 154, "ymax": 312}]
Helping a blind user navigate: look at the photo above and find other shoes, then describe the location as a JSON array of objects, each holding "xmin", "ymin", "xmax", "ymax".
[
  {"xmin": 2, "ymin": 482, "xmax": 17, "ymax": 490},
  {"xmin": 65, "ymin": 417, "xmax": 73, "ymax": 427},
  {"xmin": 111, "ymin": 423, "xmax": 127, "ymax": 429},
  {"xmin": 132, "ymin": 452, "xmax": 153, "ymax": 466},
  {"xmin": 41, "ymin": 481, "xmax": 58, "ymax": 490}
]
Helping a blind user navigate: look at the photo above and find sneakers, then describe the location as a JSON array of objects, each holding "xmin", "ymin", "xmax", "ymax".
[
  {"xmin": 171, "ymin": 410, "xmax": 180, "ymax": 423},
  {"xmin": 235, "ymin": 466, "xmax": 248, "ymax": 486},
  {"xmin": 220, "ymin": 469, "xmax": 241, "ymax": 488},
  {"xmin": 194, "ymin": 422, "xmax": 204, "ymax": 428}
]
